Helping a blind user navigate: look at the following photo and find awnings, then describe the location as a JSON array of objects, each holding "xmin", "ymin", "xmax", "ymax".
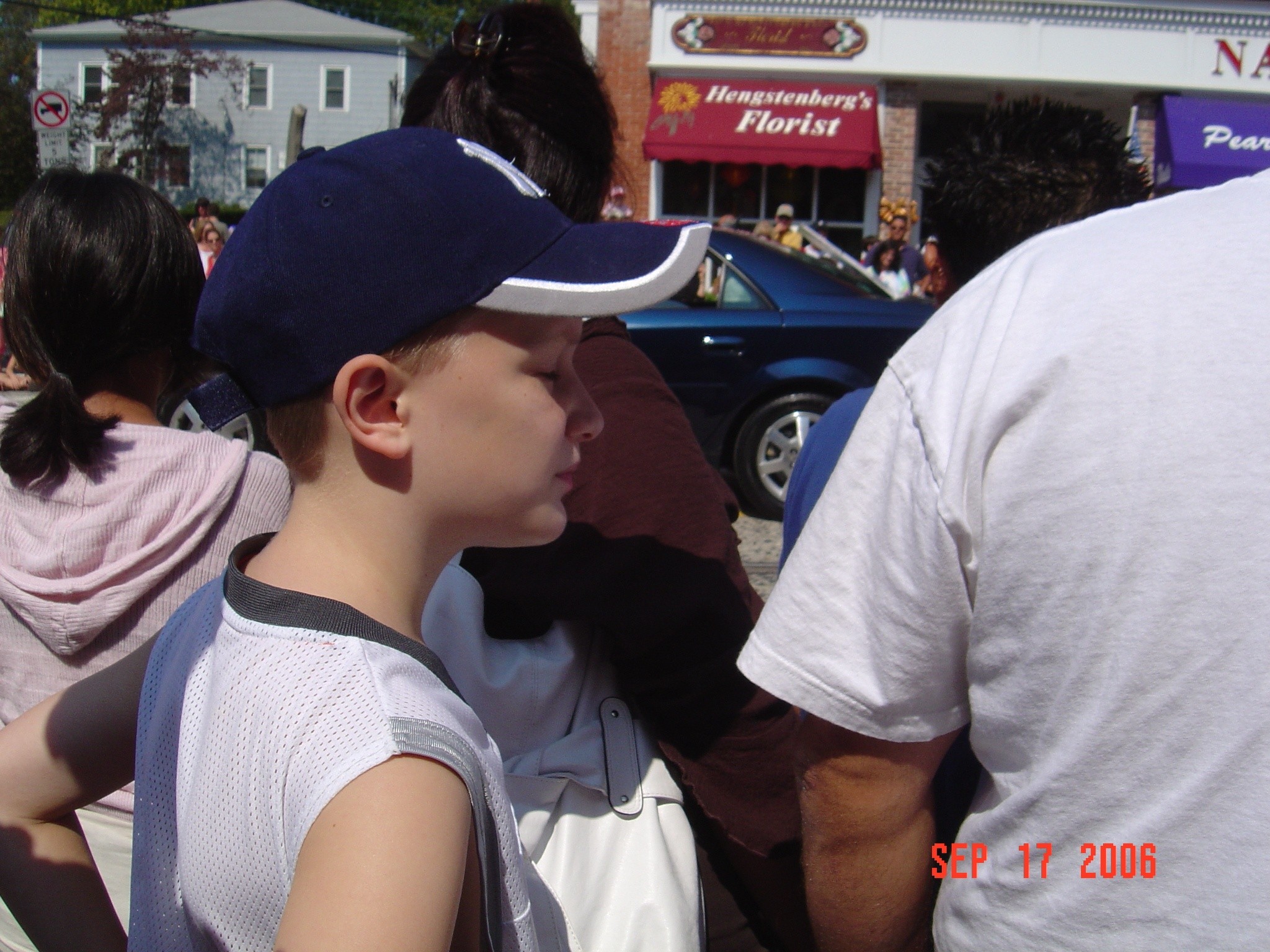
[
  {"xmin": 643, "ymin": 76, "xmax": 882, "ymax": 169},
  {"xmin": 1148, "ymin": 93, "xmax": 1270, "ymax": 189}
]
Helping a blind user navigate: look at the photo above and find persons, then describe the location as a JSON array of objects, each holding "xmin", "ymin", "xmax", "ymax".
[{"xmin": 0, "ymin": 0, "xmax": 1270, "ymax": 952}]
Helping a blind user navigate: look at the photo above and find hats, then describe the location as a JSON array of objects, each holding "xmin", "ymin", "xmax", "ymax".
[
  {"xmin": 190, "ymin": 127, "xmax": 712, "ymax": 411},
  {"xmin": 776, "ymin": 204, "xmax": 793, "ymax": 217},
  {"xmin": 196, "ymin": 196, "xmax": 208, "ymax": 205},
  {"xmin": 610, "ymin": 186, "xmax": 624, "ymax": 197}
]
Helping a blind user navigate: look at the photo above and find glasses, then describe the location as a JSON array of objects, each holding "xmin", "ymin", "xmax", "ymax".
[
  {"xmin": 208, "ymin": 237, "xmax": 221, "ymax": 243},
  {"xmin": 892, "ymin": 227, "xmax": 905, "ymax": 231}
]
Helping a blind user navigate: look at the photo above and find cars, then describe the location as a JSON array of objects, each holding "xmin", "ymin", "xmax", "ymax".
[{"xmin": 619, "ymin": 230, "xmax": 942, "ymax": 524}]
